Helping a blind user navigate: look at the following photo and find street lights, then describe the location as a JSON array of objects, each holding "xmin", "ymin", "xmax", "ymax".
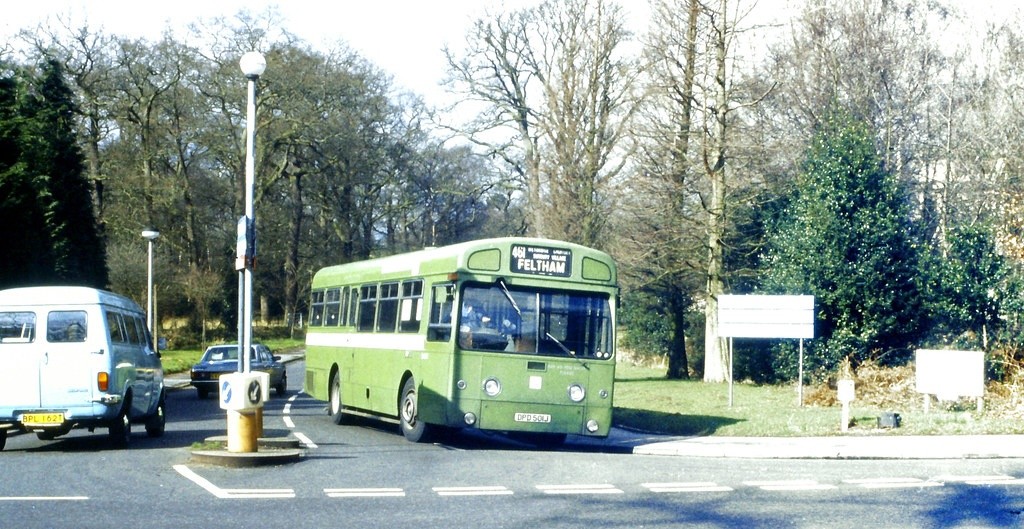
[
  {"xmin": 240, "ymin": 50, "xmax": 265, "ymax": 370},
  {"xmin": 143, "ymin": 231, "xmax": 162, "ymax": 331}
]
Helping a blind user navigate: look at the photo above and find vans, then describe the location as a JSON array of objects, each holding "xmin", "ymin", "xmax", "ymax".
[{"xmin": 0, "ymin": 287, "xmax": 165, "ymax": 450}]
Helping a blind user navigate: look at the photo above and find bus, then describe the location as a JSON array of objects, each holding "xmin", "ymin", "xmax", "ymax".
[{"xmin": 306, "ymin": 237, "xmax": 621, "ymax": 443}]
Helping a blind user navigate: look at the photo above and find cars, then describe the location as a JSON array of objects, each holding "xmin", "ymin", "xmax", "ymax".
[{"xmin": 191, "ymin": 344, "xmax": 288, "ymax": 396}]
